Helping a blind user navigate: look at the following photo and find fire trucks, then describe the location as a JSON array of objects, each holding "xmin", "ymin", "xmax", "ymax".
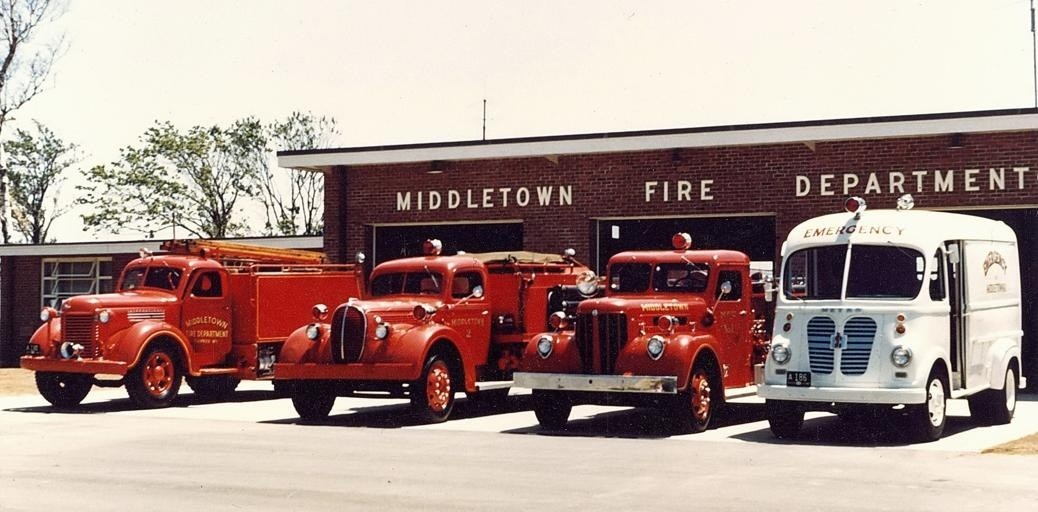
[
  {"xmin": 269, "ymin": 237, "xmax": 605, "ymax": 426},
  {"xmin": 19, "ymin": 241, "xmax": 371, "ymax": 410},
  {"xmin": 508, "ymin": 230, "xmax": 775, "ymax": 435}
]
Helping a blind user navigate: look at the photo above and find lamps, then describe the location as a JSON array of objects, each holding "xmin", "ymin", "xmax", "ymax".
[
  {"xmin": 670, "ymin": 148, "xmax": 683, "ymax": 162},
  {"xmin": 426, "ymin": 160, "xmax": 444, "ymax": 174},
  {"xmin": 946, "ymin": 133, "xmax": 965, "ymax": 149}
]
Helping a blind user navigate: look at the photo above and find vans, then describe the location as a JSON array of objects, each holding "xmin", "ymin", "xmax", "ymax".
[{"xmin": 766, "ymin": 194, "xmax": 1026, "ymax": 442}]
